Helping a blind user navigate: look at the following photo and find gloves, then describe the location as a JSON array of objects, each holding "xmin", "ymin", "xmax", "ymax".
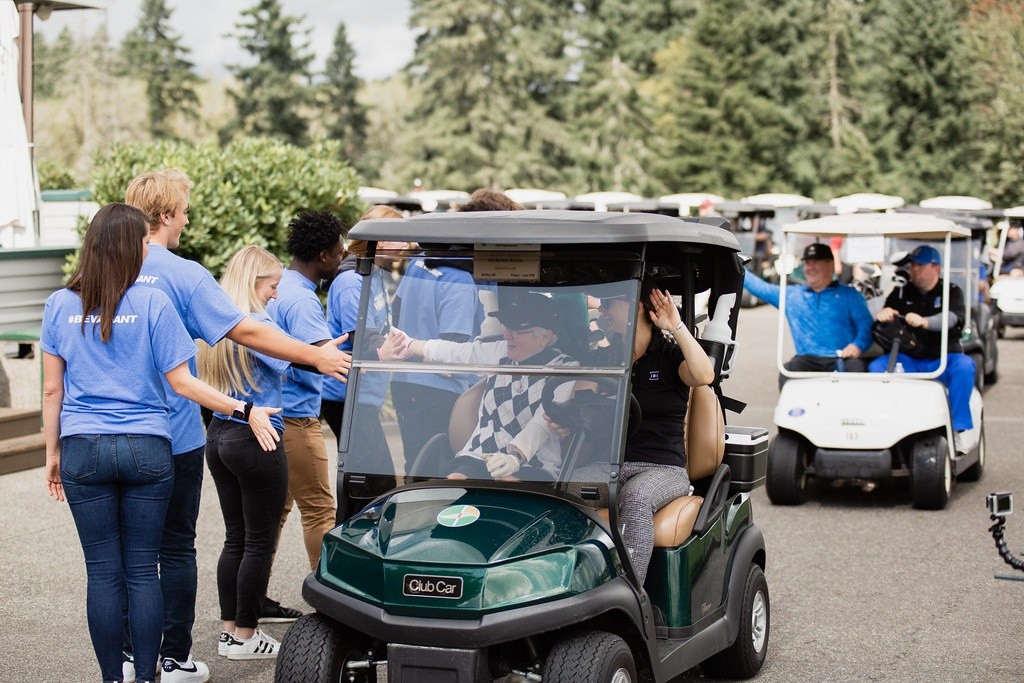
[{"xmin": 389, "ymin": 326, "xmax": 415, "ymax": 362}]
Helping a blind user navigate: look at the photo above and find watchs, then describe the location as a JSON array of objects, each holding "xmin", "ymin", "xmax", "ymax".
[
  {"xmin": 508, "ymin": 450, "xmax": 523, "ymax": 465},
  {"xmin": 232, "ymin": 400, "xmax": 247, "ymax": 420}
]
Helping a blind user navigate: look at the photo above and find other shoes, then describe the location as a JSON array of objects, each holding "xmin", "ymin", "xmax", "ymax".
[{"xmin": 953, "ymin": 430, "xmax": 967, "ymax": 456}]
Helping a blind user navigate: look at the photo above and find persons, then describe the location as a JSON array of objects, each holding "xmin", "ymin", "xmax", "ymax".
[
  {"xmin": 39, "ymin": 203, "xmax": 282, "ymax": 683},
  {"xmin": 868, "ymin": 244, "xmax": 978, "ymax": 455},
  {"xmin": 390, "ymin": 189, "xmax": 714, "ymax": 588},
  {"xmin": 696, "ymin": 200, "xmax": 1024, "ymax": 341},
  {"xmin": 123, "ymin": 172, "xmax": 406, "ymax": 682},
  {"xmin": 744, "ymin": 243, "xmax": 874, "ymax": 391}
]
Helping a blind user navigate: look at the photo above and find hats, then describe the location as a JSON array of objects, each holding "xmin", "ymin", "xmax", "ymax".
[
  {"xmin": 908, "ymin": 246, "xmax": 941, "ymax": 263},
  {"xmin": 801, "ymin": 244, "xmax": 834, "ymax": 261},
  {"xmin": 488, "ymin": 293, "xmax": 561, "ymax": 331},
  {"xmin": 582, "ymin": 274, "xmax": 652, "ymax": 299}
]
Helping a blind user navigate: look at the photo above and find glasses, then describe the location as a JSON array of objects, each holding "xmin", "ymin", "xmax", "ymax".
[
  {"xmin": 501, "ymin": 323, "xmax": 535, "ymax": 334},
  {"xmin": 601, "ymin": 299, "xmax": 630, "ymax": 307}
]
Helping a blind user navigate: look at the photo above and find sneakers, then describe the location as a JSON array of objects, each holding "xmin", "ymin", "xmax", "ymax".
[
  {"xmin": 226, "ymin": 628, "xmax": 282, "ymax": 660},
  {"xmin": 161, "ymin": 654, "xmax": 210, "ymax": 683},
  {"xmin": 257, "ymin": 596, "xmax": 303, "ymax": 624},
  {"xmin": 218, "ymin": 631, "xmax": 235, "ymax": 657},
  {"xmin": 122, "ymin": 651, "xmax": 162, "ymax": 683}
]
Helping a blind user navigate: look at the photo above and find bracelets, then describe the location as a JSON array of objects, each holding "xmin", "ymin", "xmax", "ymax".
[
  {"xmin": 228, "ymin": 397, "xmax": 233, "ymax": 420},
  {"xmin": 669, "ymin": 322, "xmax": 684, "ymax": 334},
  {"xmin": 243, "ymin": 401, "xmax": 253, "ymax": 422},
  {"xmin": 377, "ymin": 347, "xmax": 381, "ymax": 360}
]
